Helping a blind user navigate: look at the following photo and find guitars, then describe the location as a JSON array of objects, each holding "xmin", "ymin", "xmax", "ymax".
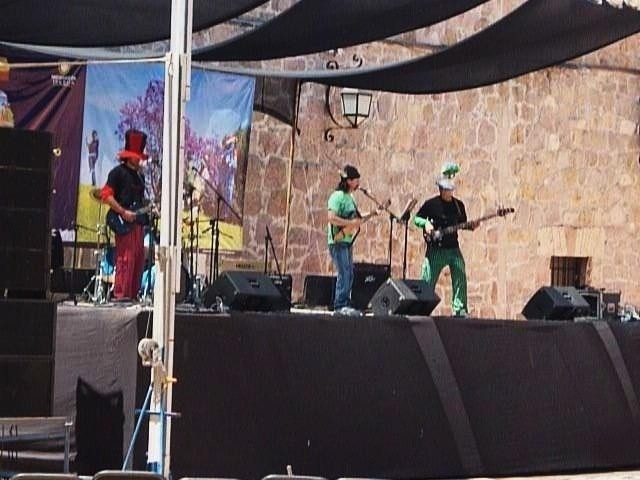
[
  {"xmin": 423, "ymin": 204, "xmax": 514, "ymax": 247},
  {"xmin": 105, "ymin": 193, "xmax": 161, "ymax": 235},
  {"xmin": 332, "ymin": 199, "xmax": 392, "ymax": 242}
]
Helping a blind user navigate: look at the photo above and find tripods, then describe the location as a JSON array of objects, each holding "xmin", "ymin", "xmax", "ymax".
[
  {"xmin": 183, "ymin": 193, "xmax": 199, "ymax": 305},
  {"xmin": 56, "ymin": 225, "xmax": 105, "ymax": 305},
  {"xmin": 78, "ymin": 200, "xmax": 108, "ymax": 305}
]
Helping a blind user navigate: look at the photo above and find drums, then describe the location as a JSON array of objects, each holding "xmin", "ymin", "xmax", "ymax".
[
  {"xmin": 141, "ymin": 263, "xmax": 191, "ymax": 307},
  {"xmin": 102, "ymin": 246, "xmax": 117, "ymax": 283}
]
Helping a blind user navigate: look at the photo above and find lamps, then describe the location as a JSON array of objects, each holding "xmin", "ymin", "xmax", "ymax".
[{"xmin": 323, "ymin": 47, "xmax": 374, "ymax": 143}]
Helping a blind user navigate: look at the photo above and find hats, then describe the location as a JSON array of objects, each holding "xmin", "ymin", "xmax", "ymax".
[
  {"xmin": 435, "ymin": 169, "xmax": 460, "ymax": 189},
  {"xmin": 117, "ymin": 129, "xmax": 150, "ymax": 161},
  {"xmin": 340, "ymin": 165, "xmax": 359, "ymax": 179}
]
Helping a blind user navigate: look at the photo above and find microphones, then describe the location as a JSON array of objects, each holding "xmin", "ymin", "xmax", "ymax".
[{"xmin": 358, "ymin": 186, "xmax": 367, "ymax": 193}]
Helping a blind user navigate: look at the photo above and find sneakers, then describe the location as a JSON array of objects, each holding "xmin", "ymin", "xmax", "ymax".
[
  {"xmin": 452, "ymin": 312, "xmax": 467, "ymax": 318},
  {"xmin": 335, "ymin": 306, "xmax": 363, "ymax": 317},
  {"xmin": 112, "ymin": 298, "xmax": 145, "ymax": 307}
]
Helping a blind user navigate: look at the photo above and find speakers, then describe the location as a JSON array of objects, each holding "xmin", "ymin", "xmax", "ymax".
[
  {"xmin": 521, "ymin": 286, "xmax": 591, "ymax": 320},
  {"xmin": 0, "ymin": 299, "xmax": 57, "ymax": 417},
  {"xmin": 349, "ymin": 263, "xmax": 391, "ymax": 310},
  {"xmin": 268, "ymin": 274, "xmax": 292, "ymax": 305},
  {"xmin": 367, "ymin": 278, "xmax": 442, "ymax": 316},
  {"xmin": 302, "ymin": 275, "xmax": 337, "ymax": 310},
  {"xmin": 204, "ymin": 270, "xmax": 282, "ymax": 311},
  {"xmin": 0, "ymin": 126, "xmax": 53, "ymax": 299}
]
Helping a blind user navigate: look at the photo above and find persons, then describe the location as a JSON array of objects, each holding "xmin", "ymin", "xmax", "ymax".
[
  {"xmin": 101, "ymin": 152, "xmax": 161, "ymax": 307},
  {"xmin": 326, "ymin": 165, "xmax": 382, "ymax": 318},
  {"xmin": 412, "ymin": 178, "xmax": 480, "ymax": 317},
  {"xmin": 85, "ymin": 130, "xmax": 99, "ymax": 188}
]
